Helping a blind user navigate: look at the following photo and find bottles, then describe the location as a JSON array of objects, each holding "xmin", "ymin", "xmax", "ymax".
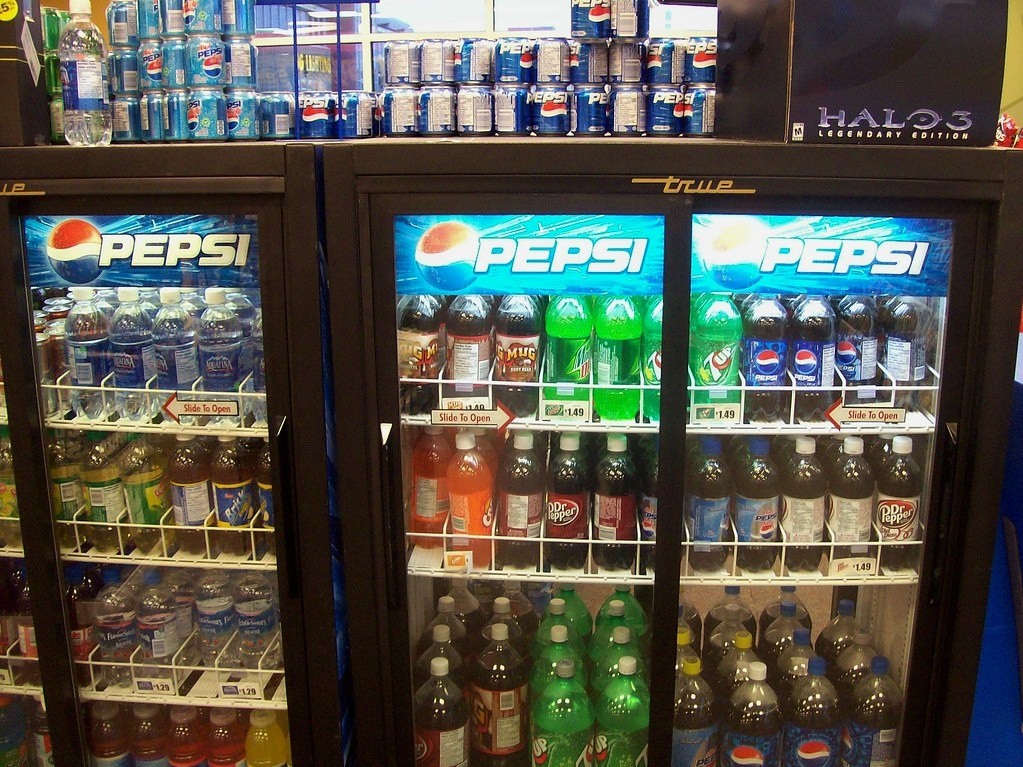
[
  {"xmin": 1, "ymin": 427, "xmax": 276, "ymax": 556},
  {"xmin": 684, "ymin": 431, "xmax": 918, "ymax": 570},
  {"xmin": 685, "ymin": 292, "xmax": 926, "ymax": 424},
  {"xmin": 395, "ymin": 294, "xmax": 663, "ymax": 422},
  {"xmin": 0, "ymin": 563, "xmax": 284, "ymax": 686},
  {"xmin": 408, "ymin": 574, "xmax": 647, "ymax": 767},
  {"xmin": 60, "ymin": 0, "xmax": 113, "ymax": 146},
  {"xmin": 671, "ymin": 583, "xmax": 902, "ymax": 767},
  {"xmin": 410, "ymin": 424, "xmax": 659, "ymax": 573},
  {"xmin": 67, "ymin": 284, "xmax": 269, "ymax": 425},
  {"xmin": 0, "ymin": 692, "xmax": 292, "ymax": 767}
]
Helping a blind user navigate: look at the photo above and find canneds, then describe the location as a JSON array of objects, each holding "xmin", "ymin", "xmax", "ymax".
[
  {"xmin": 30, "ymin": 288, "xmax": 74, "ymax": 416},
  {"xmin": 105, "ymin": 0, "xmax": 262, "ymax": 144},
  {"xmin": 40, "ymin": 6, "xmax": 73, "ymax": 145},
  {"xmin": 254, "ymin": 89, "xmax": 380, "ymax": 139},
  {"xmin": 380, "ymin": 0, "xmax": 717, "ymax": 138}
]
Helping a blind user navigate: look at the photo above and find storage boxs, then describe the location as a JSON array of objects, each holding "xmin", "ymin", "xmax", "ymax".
[{"xmin": 713, "ymin": 0, "xmax": 1011, "ymax": 148}]
